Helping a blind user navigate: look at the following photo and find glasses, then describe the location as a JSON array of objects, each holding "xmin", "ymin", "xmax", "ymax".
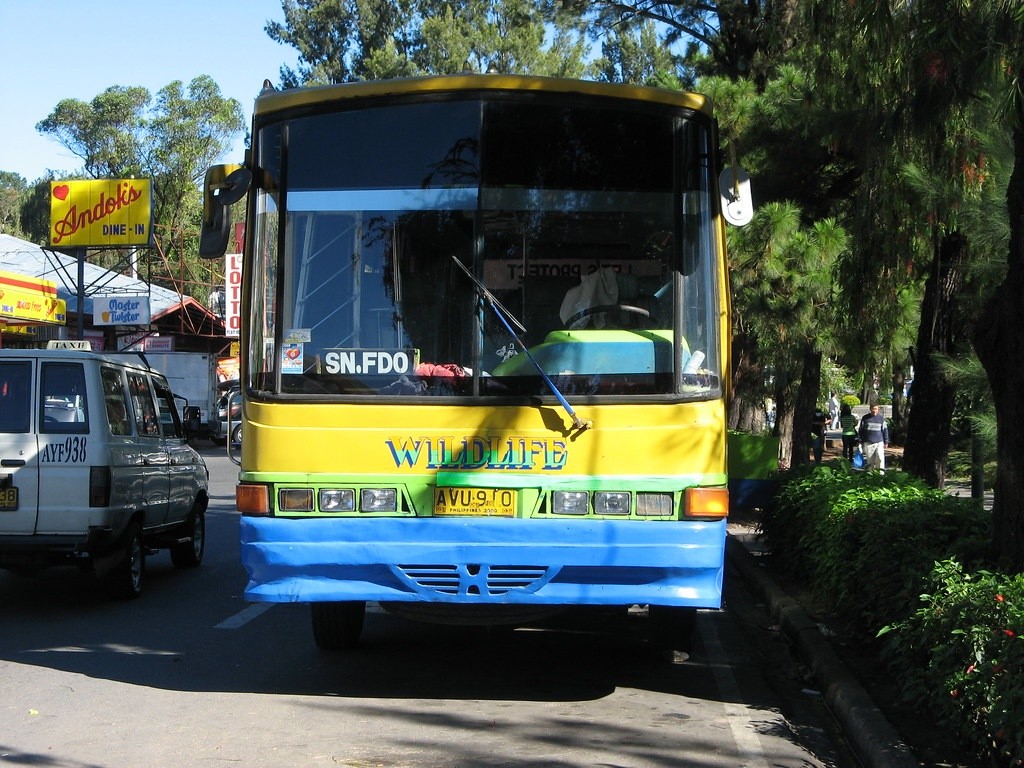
[{"xmin": 580, "ymin": 214, "xmax": 628, "ymax": 230}]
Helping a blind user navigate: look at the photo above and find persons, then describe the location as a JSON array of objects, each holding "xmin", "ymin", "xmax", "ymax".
[
  {"xmin": 838, "ymin": 405, "xmax": 858, "ymax": 461},
  {"xmin": 858, "ymin": 403, "xmax": 889, "ymax": 475},
  {"xmin": 355, "ymin": 317, "xmax": 464, "ymax": 396},
  {"xmin": 829, "ymin": 393, "xmax": 839, "ymax": 430},
  {"xmin": 810, "ymin": 408, "xmax": 832, "ymax": 461},
  {"xmin": 769, "ymin": 406, "xmax": 776, "ymax": 424}
]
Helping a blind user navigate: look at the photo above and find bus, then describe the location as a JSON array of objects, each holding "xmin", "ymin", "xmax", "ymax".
[{"xmin": 199, "ymin": 73, "xmax": 757, "ymax": 615}]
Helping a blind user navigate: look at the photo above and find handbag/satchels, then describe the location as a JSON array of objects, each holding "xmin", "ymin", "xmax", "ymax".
[{"xmin": 851, "ymin": 444, "xmax": 865, "ymax": 468}]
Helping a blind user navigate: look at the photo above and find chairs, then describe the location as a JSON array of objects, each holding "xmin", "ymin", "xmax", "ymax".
[
  {"xmin": 606, "ymin": 272, "xmax": 668, "ymax": 330},
  {"xmin": 106, "ymin": 399, "xmax": 130, "ymax": 434}
]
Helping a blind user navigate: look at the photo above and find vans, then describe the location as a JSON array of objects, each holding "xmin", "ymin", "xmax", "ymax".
[
  {"xmin": 211, "ymin": 384, "xmax": 242, "ymax": 446},
  {"xmin": 0, "ymin": 341, "xmax": 210, "ymax": 603}
]
